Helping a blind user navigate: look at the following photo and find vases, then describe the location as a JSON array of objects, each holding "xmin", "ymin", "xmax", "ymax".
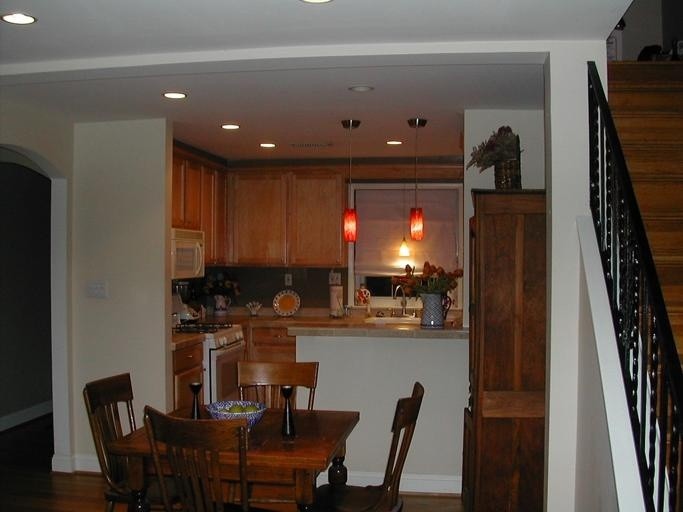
[
  {"xmin": 420, "ymin": 293, "xmax": 444, "ymax": 329},
  {"xmin": 495, "ymin": 158, "xmax": 521, "ymax": 188}
]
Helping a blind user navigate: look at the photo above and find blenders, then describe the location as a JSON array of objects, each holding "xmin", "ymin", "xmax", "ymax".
[{"xmin": 172, "ymin": 281, "xmax": 198, "ymax": 320}]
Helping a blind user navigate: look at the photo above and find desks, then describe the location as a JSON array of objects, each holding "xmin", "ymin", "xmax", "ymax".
[{"xmin": 110, "ymin": 404, "xmax": 362, "ymax": 509}]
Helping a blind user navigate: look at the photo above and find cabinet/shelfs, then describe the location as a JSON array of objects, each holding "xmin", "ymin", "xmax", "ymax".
[
  {"xmin": 461, "ymin": 188, "xmax": 546, "ymax": 512},
  {"xmin": 172, "ymin": 333, "xmax": 206, "ymax": 410},
  {"xmin": 251, "ymin": 327, "xmax": 296, "ymax": 407},
  {"xmin": 172, "ymin": 145, "xmax": 348, "ymax": 268}
]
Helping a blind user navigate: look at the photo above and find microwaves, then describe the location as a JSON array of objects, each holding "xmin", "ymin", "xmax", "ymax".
[{"xmin": 170, "ymin": 238, "xmax": 204, "ymax": 281}]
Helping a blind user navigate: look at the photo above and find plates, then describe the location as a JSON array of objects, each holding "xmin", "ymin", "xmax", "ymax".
[{"xmin": 273, "ymin": 290, "xmax": 299, "ymax": 317}]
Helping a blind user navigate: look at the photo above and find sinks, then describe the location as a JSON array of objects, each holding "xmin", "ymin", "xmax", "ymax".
[{"xmin": 366, "ymin": 317, "xmax": 421, "ymax": 324}]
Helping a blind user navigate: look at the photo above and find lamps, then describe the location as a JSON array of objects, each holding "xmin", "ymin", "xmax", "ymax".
[
  {"xmin": 408, "ymin": 119, "xmax": 427, "ymax": 241},
  {"xmin": 398, "ymin": 189, "xmax": 408, "ymax": 256},
  {"xmin": 342, "ymin": 119, "xmax": 361, "ymax": 243}
]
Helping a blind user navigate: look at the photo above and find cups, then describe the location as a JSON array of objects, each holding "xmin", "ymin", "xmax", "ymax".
[
  {"xmin": 343, "ymin": 304, "xmax": 352, "ymax": 318},
  {"xmin": 205, "ymin": 304, "xmax": 214, "ymax": 317}
]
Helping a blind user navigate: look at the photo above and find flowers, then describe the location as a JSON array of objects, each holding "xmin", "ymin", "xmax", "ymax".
[
  {"xmin": 466, "ymin": 126, "xmax": 525, "ymax": 174},
  {"xmin": 391, "ymin": 261, "xmax": 464, "ymax": 301}
]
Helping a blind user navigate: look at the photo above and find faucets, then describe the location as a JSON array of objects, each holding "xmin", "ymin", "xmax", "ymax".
[{"xmin": 393, "ymin": 285, "xmax": 407, "ymax": 316}]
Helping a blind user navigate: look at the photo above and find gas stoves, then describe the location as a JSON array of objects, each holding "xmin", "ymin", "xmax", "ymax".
[{"xmin": 174, "ymin": 318, "xmax": 243, "ymax": 349}]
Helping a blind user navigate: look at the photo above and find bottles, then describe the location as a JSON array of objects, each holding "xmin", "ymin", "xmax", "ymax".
[
  {"xmin": 356, "ymin": 284, "xmax": 370, "ymax": 315},
  {"xmin": 188, "ymin": 382, "xmax": 202, "ymax": 419},
  {"xmin": 280, "ymin": 385, "xmax": 295, "ymax": 438}
]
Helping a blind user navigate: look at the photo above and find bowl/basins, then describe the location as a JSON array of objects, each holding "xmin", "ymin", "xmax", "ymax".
[{"xmin": 204, "ymin": 401, "xmax": 266, "ymax": 429}]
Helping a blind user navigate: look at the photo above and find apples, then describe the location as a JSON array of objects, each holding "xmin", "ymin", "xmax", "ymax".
[
  {"xmin": 244, "ymin": 405, "xmax": 258, "ymax": 412},
  {"xmin": 228, "ymin": 405, "xmax": 243, "ymax": 413},
  {"xmin": 218, "ymin": 409, "xmax": 228, "ymax": 412}
]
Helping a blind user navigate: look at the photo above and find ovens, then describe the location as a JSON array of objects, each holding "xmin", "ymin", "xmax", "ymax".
[{"xmin": 209, "ymin": 340, "xmax": 247, "ymax": 402}]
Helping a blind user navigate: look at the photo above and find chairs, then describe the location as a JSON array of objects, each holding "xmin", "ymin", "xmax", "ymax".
[
  {"xmin": 315, "ymin": 381, "xmax": 424, "ymax": 511},
  {"xmin": 142, "ymin": 405, "xmax": 250, "ymax": 509},
  {"xmin": 234, "ymin": 363, "xmax": 323, "ymax": 408},
  {"xmin": 84, "ymin": 373, "xmax": 196, "ymax": 508}
]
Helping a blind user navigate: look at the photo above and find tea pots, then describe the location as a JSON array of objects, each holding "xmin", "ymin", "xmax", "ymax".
[
  {"xmin": 419, "ymin": 293, "xmax": 452, "ymax": 329},
  {"xmin": 213, "ymin": 295, "xmax": 231, "ymax": 315}
]
